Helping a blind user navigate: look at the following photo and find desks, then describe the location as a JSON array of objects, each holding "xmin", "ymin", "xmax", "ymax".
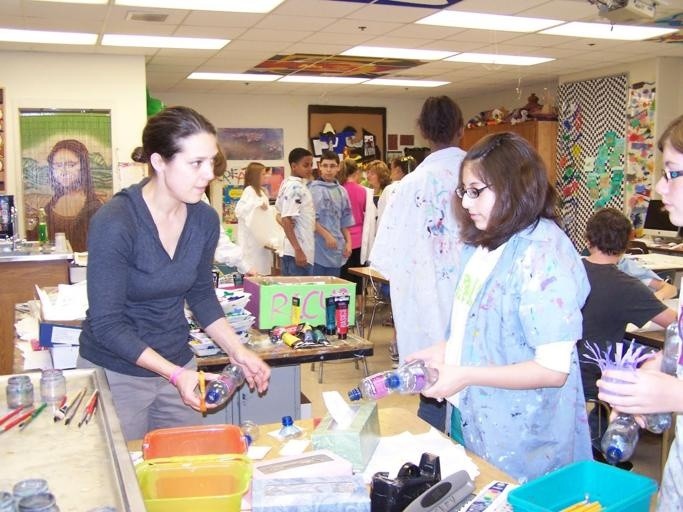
[
  {"xmin": 627, "ymin": 298, "xmax": 680, "ymax": 346},
  {"xmin": 347, "ymin": 266, "xmax": 391, "ymax": 346},
  {"xmin": 127, "ymin": 404, "xmax": 520, "ymax": 512},
  {"xmin": 196, "ymin": 332, "xmax": 372, "ymax": 395},
  {"xmin": 627, "ymin": 253, "xmax": 683, "ymax": 283}
]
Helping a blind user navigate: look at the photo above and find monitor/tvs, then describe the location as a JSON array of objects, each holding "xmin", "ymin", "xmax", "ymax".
[{"xmin": 642, "ymin": 200, "xmax": 680, "ymax": 243}]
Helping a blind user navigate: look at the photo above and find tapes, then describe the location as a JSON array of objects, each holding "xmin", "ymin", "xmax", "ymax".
[{"xmin": 397, "ymin": 463, "xmax": 421, "ymax": 478}]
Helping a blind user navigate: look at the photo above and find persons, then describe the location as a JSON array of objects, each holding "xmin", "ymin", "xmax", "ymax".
[
  {"xmin": 76, "ymin": 96, "xmax": 683, "ymax": 512},
  {"xmin": 26, "ymin": 140, "xmax": 103, "ymax": 253}
]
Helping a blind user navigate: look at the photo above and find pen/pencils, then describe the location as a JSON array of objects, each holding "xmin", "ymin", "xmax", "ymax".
[
  {"xmin": 559, "ymin": 500, "xmax": 606, "ymax": 512},
  {"xmin": 198, "ymin": 370, "xmax": 207, "ymax": 413}
]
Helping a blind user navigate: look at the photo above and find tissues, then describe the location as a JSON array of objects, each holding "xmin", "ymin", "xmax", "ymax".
[{"xmin": 312, "ymin": 389, "xmax": 380, "ymax": 473}]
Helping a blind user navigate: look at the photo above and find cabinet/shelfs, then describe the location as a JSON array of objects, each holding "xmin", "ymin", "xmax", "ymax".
[
  {"xmin": 463, "ymin": 121, "xmax": 558, "ymax": 184},
  {"xmin": 1, "ymin": 251, "xmax": 75, "ymax": 375}
]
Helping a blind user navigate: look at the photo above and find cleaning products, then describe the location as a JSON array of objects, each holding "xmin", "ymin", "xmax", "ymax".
[{"xmin": 37, "ymin": 207, "xmax": 48, "ymax": 251}]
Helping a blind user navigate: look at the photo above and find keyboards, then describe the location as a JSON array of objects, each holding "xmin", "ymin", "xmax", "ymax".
[{"xmin": 634, "ymin": 241, "xmax": 661, "ymax": 248}]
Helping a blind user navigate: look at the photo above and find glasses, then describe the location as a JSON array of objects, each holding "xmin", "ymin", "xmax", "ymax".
[
  {"xmin": 661, "ymin": 166, "xmax": 683, "ymax": 182},
  {"xmin": 454, "ymin": 185, "xmax": 489, "ymax": 198}
]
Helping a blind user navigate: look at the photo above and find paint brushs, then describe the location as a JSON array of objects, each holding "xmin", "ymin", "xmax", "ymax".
[
  {"xmin": 0, "ymin": 403, "xmax": 47, "ymax": 434},
  {"xmin": 54, "ymin": 387, "xmax": 100, "ymax": 427}
]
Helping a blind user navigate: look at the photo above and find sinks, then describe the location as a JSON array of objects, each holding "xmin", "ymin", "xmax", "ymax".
[{"xmin": 0, "ymin": 243, "xmax": 50, "ymax": 256}]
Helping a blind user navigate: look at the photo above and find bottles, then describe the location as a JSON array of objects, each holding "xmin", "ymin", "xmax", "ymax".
[
  {"xmin": 34, "ymin": 208, "xmax": 49, "ymax": 250},
  {"xmin": 40, "ymin": 369, "xmax": 67, "ymax": 401},
  {"xmin": 600, "ymin": 369, "xmax": 672, "ymax": 465},
  {"xmin": 1, "ymin": 478, "xmax": 62, "ymax": 512},
  {"xmin": 660, "ymin": 320, "xmax": 682, "ymax": 375},
  {"xmin": 279, "ymin": 415, "xmax": 300, "ymax": 441},
  {"xmin": 345, "ymin": 360, "xmax": 440, "ymax": 403},
  {"xmin": 6, "ymin": 376, "xmax": 34, "ymax": 407},
  {"xmin": 55, "ymin": 232, "xmax": 66, "ymax": 252},
  {"xmin": 241, "ymin": 419, "xmax": 257, "ymax": 445},
  {"xmin": 205, "ymin": 364, "xmax": 244, "ymax": 404}
]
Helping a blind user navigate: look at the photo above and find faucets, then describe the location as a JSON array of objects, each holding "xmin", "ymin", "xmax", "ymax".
[{"xmin": 10, "ymin": 206, "xmax": 22, "ymax": 251}]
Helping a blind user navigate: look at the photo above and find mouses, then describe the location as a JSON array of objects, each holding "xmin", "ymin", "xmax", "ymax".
[{"xmin": 667, "ymin": 242, "xmax": 678, "ymax": 247}]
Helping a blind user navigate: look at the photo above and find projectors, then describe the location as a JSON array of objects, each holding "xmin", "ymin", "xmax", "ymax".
[{"xmin": 599, "ymin": 0, "xmax": 655, "ymax": 23}]
